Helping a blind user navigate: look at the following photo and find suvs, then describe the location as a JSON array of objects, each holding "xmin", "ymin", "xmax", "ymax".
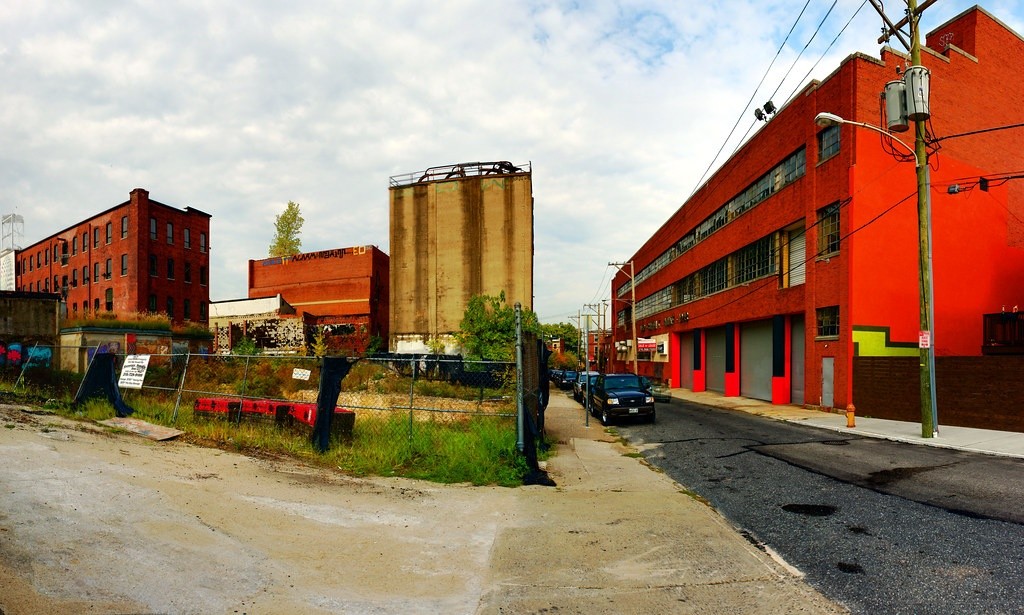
[{"xmin": 590, "ymin": 372, "xmax": 656, "ymax": 426}]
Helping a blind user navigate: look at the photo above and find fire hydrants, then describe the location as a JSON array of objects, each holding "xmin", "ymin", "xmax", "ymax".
[{"xmin": 844, "ymin": 403, "xmax": 856, "ymax": 428}]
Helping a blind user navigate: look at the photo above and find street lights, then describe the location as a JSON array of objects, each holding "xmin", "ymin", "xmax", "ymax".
[
  {"xmin": 815, "ymin": 112, "xmax": 937, "ymax": 440},
  {"xmin": 601, "ymin": 299, "xmax": 638, "ymax": 375}
]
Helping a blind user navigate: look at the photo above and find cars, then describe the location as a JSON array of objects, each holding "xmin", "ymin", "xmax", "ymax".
[
  {"xmin": 550, "ymin": 369, "xmax": 600, "ymax": 405},
  {"xmin": 640, "ymin": 375, "xmax": 672, "ymax": 402}
]
